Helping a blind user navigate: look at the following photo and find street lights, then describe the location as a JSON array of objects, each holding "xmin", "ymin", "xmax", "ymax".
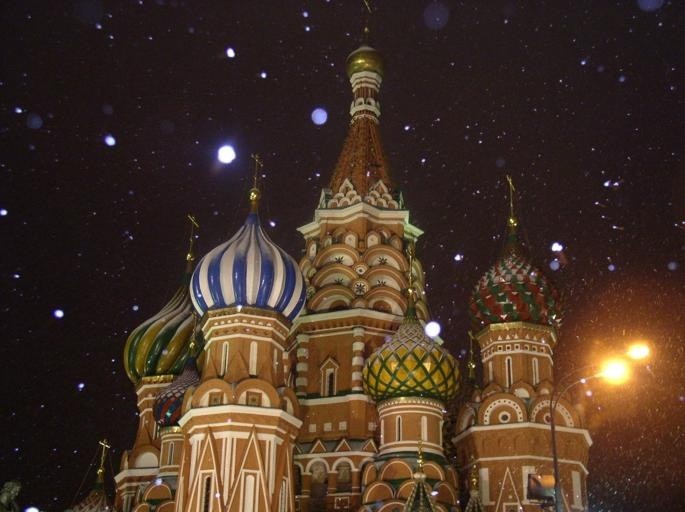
[{"xmin": 549, "ymin": 341, "xmax": 651, "ymax": 512}]
